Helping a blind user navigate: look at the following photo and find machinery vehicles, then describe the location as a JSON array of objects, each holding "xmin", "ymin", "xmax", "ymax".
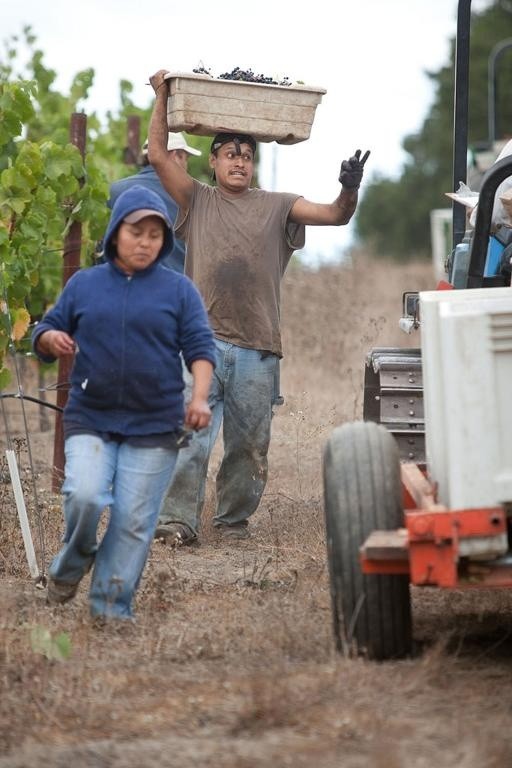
[{"xmin": 322, "ymin": 0, "xmax": 512, "ymax": 661}]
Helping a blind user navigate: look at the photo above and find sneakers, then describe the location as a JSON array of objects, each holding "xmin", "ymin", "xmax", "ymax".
[
  {"xmin": 46, "ymin": 578, "xmax": 76, "ymax": 604},
  {"xmin": 95, "ymin": 615, "xmax": 130, "ymax": 633},
  {"xmin": 154, "ymin": 523, "xmax": 196, "ymax": 546},
  {"xmin": 218, "ymin": 523, "xmax": 248, "ymax": 540}
]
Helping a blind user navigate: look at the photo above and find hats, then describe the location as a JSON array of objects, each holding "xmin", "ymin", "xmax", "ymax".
[
  {"xmin": 142, "ymin": 132, "xmax": 201, "ymax": 160},
  {"xmin": 123, "ymin": 209, "xmax": 169, "ymax": 230},
  {"xmin": 210, "ymin": 132, "xmax": 256, "ymax": 180}
]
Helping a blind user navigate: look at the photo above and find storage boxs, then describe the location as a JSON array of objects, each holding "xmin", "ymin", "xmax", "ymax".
[{"xmin": 161, "ymin": 72, "xmax": 327, "ymax": 146}]
